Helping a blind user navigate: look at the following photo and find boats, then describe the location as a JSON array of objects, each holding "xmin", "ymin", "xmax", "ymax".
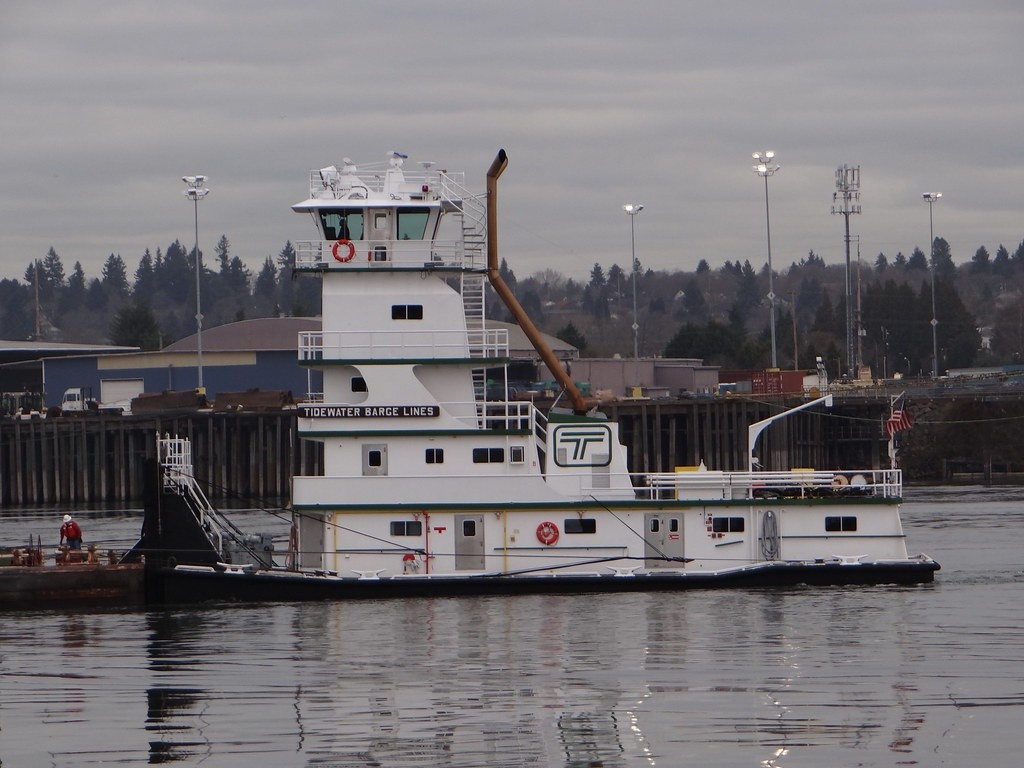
[{"xmin": 1, "ymin": 149, "xmax": 943, "ymax": 605}]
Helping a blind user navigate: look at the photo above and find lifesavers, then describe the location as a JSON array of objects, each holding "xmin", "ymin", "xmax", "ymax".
[
  {"xmin": 403, "ymin": 554, "xmax": 415, "ymax": 566},
  {"xmin": 537, "ymin": 521, "xmax": 559, "ymax": 544},
  {"xmin": 264, "ymin": 546, "xmax": 270, "ymax": 551},
  {"xmin": 332, "ymin": 239, "xmax": 355, "ymax": 264}
]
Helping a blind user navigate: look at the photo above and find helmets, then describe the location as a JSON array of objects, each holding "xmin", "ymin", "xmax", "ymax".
[{"xmin": 63, "ymin": 515, "xmax": 72, "ymax": 523}]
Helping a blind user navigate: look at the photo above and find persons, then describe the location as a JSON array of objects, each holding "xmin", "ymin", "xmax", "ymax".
[
  {"xmin": 60, "ymin": 515, "xmax": 83, "ymax": 549},
  {"xmin": 338, "ymin": 219, "xmax": 350, "ymax": 240}
]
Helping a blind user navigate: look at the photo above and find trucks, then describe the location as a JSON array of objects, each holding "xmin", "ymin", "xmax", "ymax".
[{"xmin": 61, "ymin": 387, "xmax": 132, "ymax": 414}]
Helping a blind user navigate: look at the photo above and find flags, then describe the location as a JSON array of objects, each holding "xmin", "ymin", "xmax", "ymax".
[{"xmin": 887, "ymin": 395, "xmax": 912, "ymax": 436}]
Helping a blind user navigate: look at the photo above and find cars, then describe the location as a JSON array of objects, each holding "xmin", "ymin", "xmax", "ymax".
[{"xmin": 681, "ymin": 390, "xmax": 698, "ymax": 398}]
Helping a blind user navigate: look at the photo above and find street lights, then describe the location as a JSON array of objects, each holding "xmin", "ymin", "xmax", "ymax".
[
  {"xmin": 622, "ymin": 202, "xmax": 644, "ymax": 356},
  {"xmin": 923, "ymin": 191, "xmax": 943, "ymax": 377},
  {"xmin": 752, "ymin": 150, "xmax": 782, "ymax": 367},
  {"xmin": 181, "ymin": 173, "xmax": 213, "ymax": 387}
]
